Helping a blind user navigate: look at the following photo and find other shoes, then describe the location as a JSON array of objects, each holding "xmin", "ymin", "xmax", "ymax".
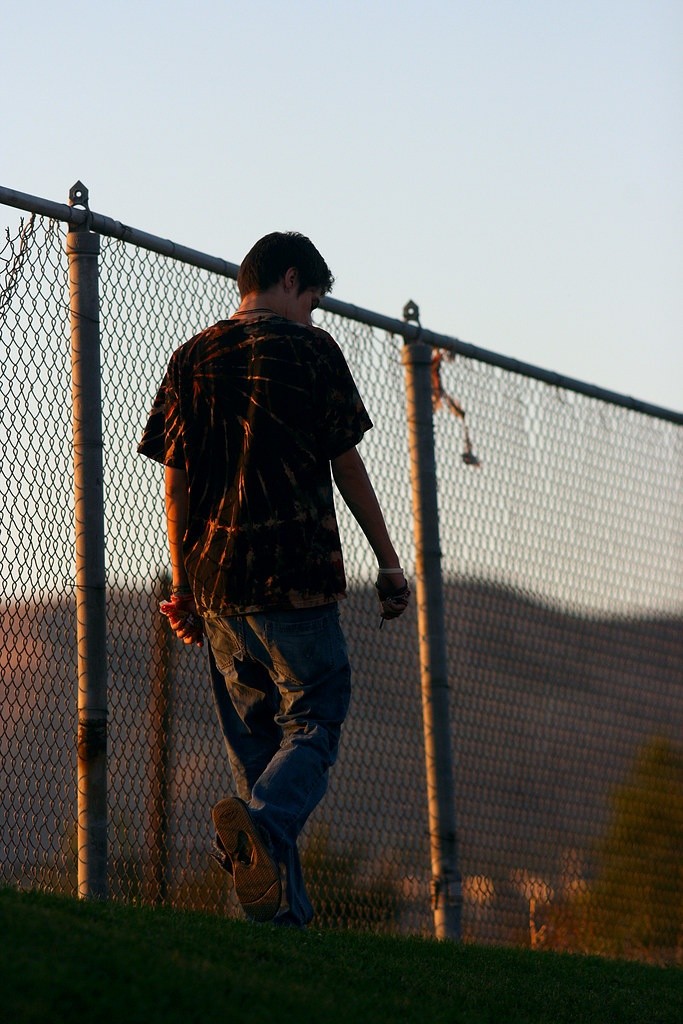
[{"xmin": 212, "ymin": 797, "xmax": 282, "ymax": 921}]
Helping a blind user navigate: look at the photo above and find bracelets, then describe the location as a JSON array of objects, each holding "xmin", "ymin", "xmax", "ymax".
[
  {"xmin": 379, "ymin": 568, "xmax": 403, "ymax": 573},
  {"xmin": 171, "ymin": 585, "xmax": 192, "ymax": 602}
]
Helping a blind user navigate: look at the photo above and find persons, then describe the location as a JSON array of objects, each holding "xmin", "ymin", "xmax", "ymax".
[{"xmin": 136, "ymin": 232, "xmax": 408, "ymax": 922}]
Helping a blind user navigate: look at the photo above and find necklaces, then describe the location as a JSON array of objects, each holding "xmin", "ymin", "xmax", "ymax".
[{"xmin": 231, "ymin": 308, "xmax": 278, "ymax": 314}]
